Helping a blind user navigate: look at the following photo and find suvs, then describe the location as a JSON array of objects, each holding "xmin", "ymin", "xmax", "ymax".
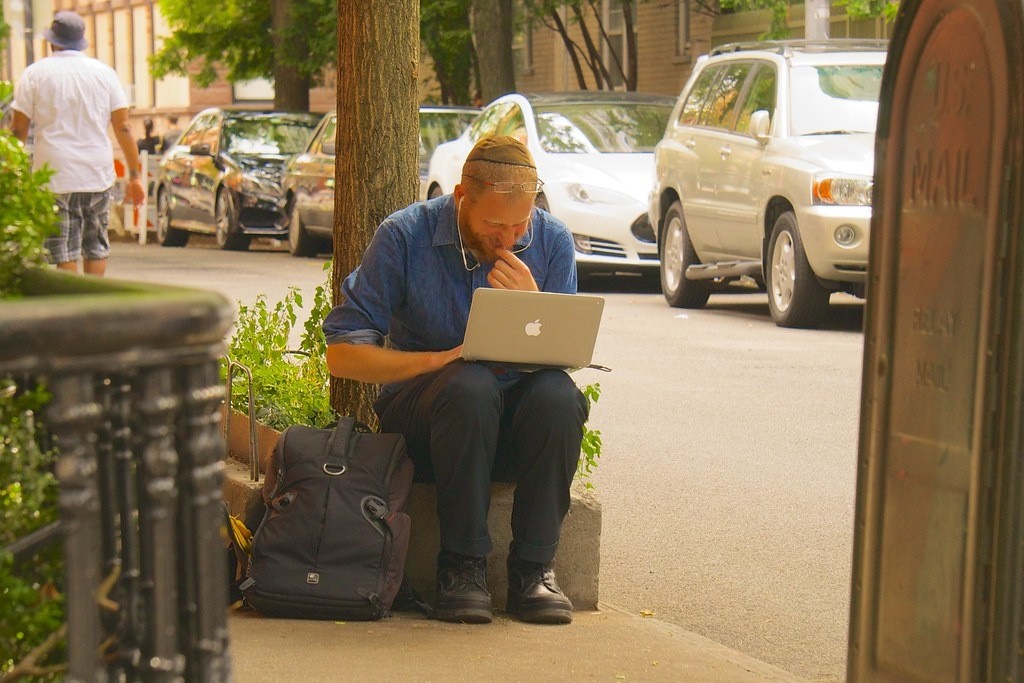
[{"xmin": 649, "ymin": 38, "xmax": 890, "ymax": 328}]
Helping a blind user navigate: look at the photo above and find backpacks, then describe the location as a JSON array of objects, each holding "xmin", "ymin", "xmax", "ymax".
[{"xmin": 221, "ymin": 417, "xmax": 415, "ymax": 622}]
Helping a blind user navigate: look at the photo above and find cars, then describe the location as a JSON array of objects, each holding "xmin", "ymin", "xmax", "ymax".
[{"xmin": 154, "ymin": 92, "xmax": 679, "ymax": 266}]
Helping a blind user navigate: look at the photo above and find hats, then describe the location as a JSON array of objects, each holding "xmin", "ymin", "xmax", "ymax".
[
  {"xmin": 41, "ymin": 12, "xmax": 89, "ymax": 50},
  {"xmin": 466, "ymin": 135, "xmax": 537, "ymax": 169}
]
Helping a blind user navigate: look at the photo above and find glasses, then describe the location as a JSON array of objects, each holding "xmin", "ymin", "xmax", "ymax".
[{"xmin": 462, "ymin": 174, "xmax": 545, "ymax": 194}]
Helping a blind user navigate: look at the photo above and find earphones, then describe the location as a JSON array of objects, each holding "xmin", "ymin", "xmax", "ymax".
[{"xmin": 458, "ymin": 195, "xmax": 465, "ymax": 210}]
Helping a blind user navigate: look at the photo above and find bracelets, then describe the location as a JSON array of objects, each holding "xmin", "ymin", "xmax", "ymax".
[{"xmin": 128, "ymin": 169, "xmax": 139, "ymax": 177}]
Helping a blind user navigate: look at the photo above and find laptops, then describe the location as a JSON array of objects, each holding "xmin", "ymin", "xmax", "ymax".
[{"xmin": 446, "ymin": 287, "xmax": 606, "ymax": 373}]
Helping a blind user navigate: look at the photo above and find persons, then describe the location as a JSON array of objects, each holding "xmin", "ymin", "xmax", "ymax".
[
  {"xmin": 7, "ymin": 10, "xmax": 146, "ymax": 277},
  {"xmin": 136, "ymin": 112, "xmax": 187, "ymax": 154},
  {"xmin": 320, "ymin": 133, "xmax": 590, "ymax": 625}
]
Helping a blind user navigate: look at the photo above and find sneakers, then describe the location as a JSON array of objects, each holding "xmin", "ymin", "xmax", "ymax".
[
  {"xmin": 506, "ymin": 554, "xmax": 574, "ymax": 623},
  {"xmin": 424, "ymin": 551, "xmax": 494, "ymax": 622}
]
